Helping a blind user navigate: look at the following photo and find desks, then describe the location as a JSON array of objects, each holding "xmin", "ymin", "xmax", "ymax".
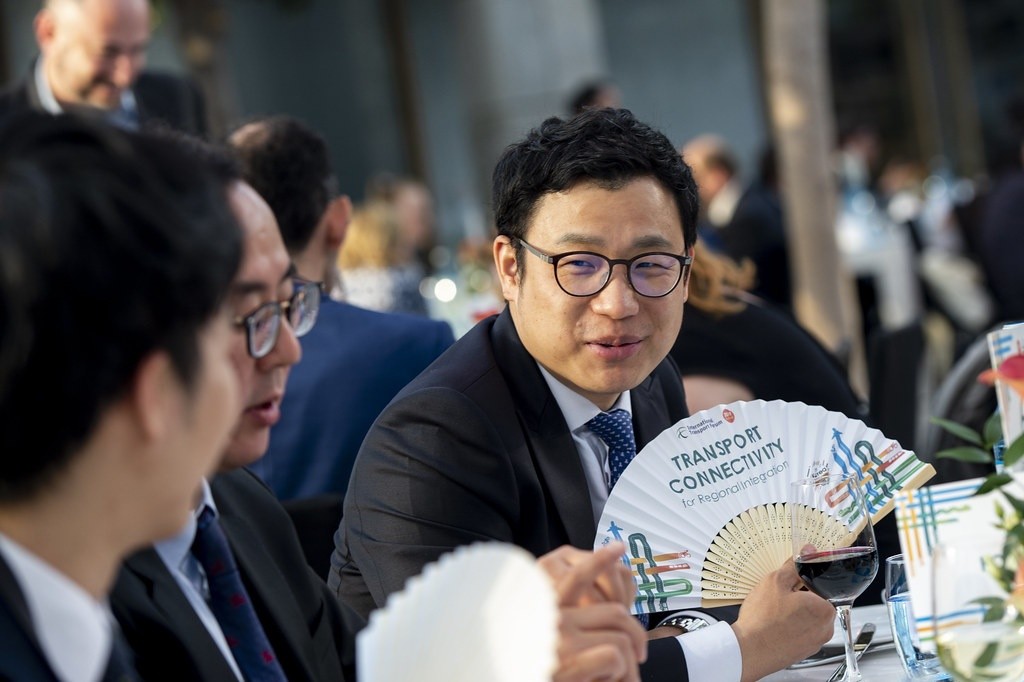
[{"xmin": 752, "ymin": 603, "xmax": 911, "ymax": 682}]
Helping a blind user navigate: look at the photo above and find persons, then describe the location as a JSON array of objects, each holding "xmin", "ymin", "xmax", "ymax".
[
  {"xmin": 0, "ymin": 0, "xmax": 1024, "ymax": 682},
  {"xmin": 328, "ymin": 105, "xmax": 836, "ymax": 682}
]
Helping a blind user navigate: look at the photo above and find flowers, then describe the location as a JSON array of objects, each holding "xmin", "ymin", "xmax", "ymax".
[{"xmin": 930, "ymin": 348, "xmax": 1023, "ymax": 622}]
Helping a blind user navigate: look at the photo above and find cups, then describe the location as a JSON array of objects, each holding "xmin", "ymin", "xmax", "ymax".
[
  {"xmin": 992, "ymin": 439, "xmax": 1005, "ymax": 475},
  {"xmin": 885, "ymin": 554, "xmax": 954, "ymax": 682}
]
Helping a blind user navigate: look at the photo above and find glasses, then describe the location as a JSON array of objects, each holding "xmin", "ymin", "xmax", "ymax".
[
  {"xmin": 230, "ymin": 276, "xmax": 326, "ymax": 358},
  {"xmin": 512, "ymin": 235, "xmax": 691, "ymax": 298}
]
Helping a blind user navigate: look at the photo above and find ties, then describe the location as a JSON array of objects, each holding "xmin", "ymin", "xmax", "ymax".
[
  {"xmin": 906, "ymin": 220, "xmax": 933, "ymax": 309},
  {"xmin": 191, "ymin": 508, "xmax": 288, "ymax": 682},
  {"xmin": 585, "ymin": 409, "xmax": 650, "ymax": 628},
  {"xmin": 103, "ymin": 625, "xmax": 142, "ymax": 682}
]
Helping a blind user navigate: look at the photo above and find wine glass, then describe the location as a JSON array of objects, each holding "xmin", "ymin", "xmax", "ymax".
[{"xmin": 791, "ymin": 473, "xmax": 880, "ymax": 682}]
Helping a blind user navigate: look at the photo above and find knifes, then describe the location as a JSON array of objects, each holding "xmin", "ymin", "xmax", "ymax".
[{"xmin": 826, "ymin": 623, "xmax": 876, "ymax": 682}]
